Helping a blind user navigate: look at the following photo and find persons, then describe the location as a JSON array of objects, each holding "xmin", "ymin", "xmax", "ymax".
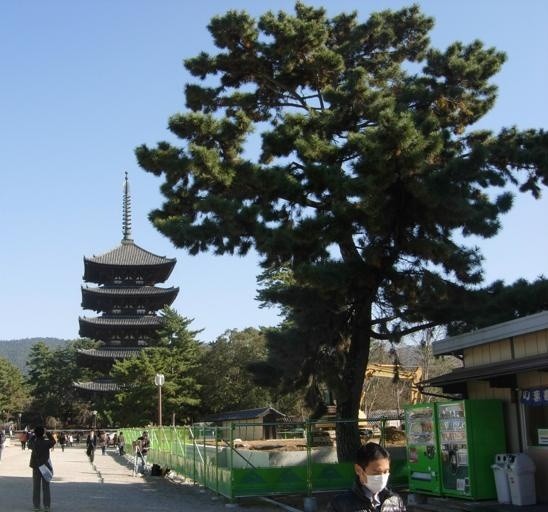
[
  {"xmin": 323, "ymin": 442, "xmax": 406, "ymax": 511},
  {"xmin": 0, "ymin": 427, "xmax": 127, "ymax": 463},
  {"xmin": 28, "ymin": 425, "xmax": 54, "ymax": 511},
  {"xmin": 133, "ymin": 429, "xmax": 152, "ymax": 474}
]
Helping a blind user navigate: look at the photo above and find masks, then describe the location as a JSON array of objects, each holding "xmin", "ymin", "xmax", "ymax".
[{"xmin": 357, "ymin": 467, "xmax": 390, "ymax": 493}]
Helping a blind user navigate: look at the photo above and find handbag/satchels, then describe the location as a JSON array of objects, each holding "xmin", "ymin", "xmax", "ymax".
[{"xmin": 39, "ymin": 464, "xmax": 53, "ymax": 484}]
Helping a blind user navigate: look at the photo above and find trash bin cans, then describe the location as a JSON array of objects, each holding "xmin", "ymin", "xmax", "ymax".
[{"xmin": 490, "ymin": 453, "xmax": 536, "ymax": 506}]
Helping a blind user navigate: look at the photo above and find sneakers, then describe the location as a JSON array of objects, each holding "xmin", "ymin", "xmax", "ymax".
[{"xmin": 32, "ymin": 505, "xmax": 51, "ymax": 512}]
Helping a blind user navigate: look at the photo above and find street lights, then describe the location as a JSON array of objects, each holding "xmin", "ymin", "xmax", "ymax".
[
  {"xmin": 18, "ymin": 412, "xmax": 22, "ymax": 430},
  {"xmin": 91, "ymin": 409, "xmax": 97, "ymax": 429},
  {"xmin": 153, "ymin": 373, "xmax": 165, "ymax": 429}
]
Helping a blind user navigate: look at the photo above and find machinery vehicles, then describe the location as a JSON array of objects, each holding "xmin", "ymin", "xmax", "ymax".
[{"xmin": 308, "ymin": 360, "xmax": 426, "ymax": 446}]
